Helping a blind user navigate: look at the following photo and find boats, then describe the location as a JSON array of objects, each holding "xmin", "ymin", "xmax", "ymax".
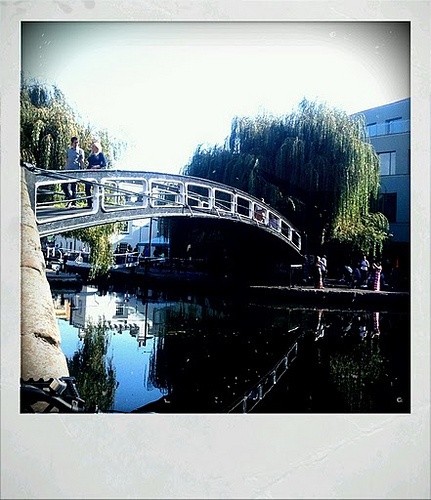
[{"xmin": 47, "ymin": 270, "xmax": 83, "ymax": 292}]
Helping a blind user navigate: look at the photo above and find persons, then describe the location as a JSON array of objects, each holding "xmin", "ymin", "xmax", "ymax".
[
  {"xmin": 304, "ymin": 255, "xmax": 382, "ymax": 292},
  {"xmin": 255, "ymin": 198, "xmax": 269, "ymax": 221},
  {"xmin": 61, "ymin": 137, "xmax": 85, "ymax": 208},
  {"xmin": 80, "ymin": 245, "xmax": 166, "ymax": 268},
  {"xmin": 335, "ymin": 310, "xmax": 381, "ymax": 343},
  {"xmin": 270, "ymin": 214, "xmax": 277, "ymax": 229},
  {"xmin": 83, "ymin": 143, "xmax": 107, "ymax": 209}
]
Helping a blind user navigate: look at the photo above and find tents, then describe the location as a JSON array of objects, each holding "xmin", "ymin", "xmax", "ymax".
[{"xmin": 137, "ymin": 235, "xmax": 169, "ymax": 246}]
